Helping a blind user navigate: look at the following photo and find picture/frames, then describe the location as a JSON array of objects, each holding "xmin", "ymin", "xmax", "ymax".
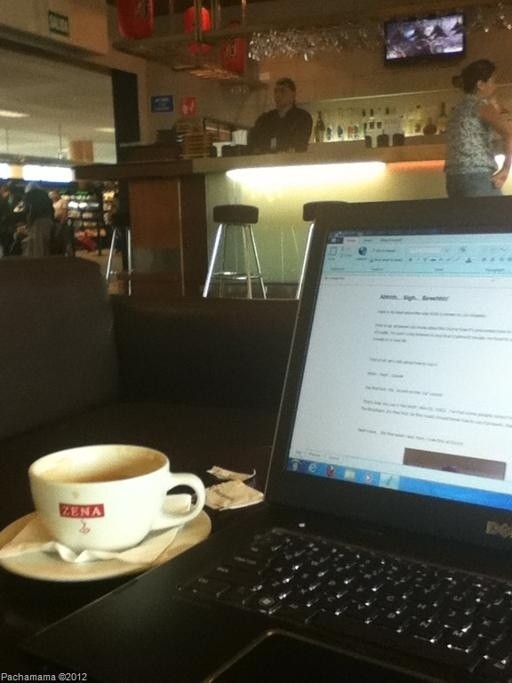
[{"xmin": 151, "ymin": 95, "xmax": 172, "ymax": 113}]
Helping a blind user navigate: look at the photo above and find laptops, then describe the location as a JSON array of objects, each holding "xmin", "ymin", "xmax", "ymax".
[{"xmin": 15, "ymin": 193, "xmax": 512, "ymax": 683}]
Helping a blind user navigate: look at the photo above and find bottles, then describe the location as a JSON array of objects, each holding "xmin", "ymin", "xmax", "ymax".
[{"xmin": 313, "ymin": 99, "xmax": 456, "ymax": 142}]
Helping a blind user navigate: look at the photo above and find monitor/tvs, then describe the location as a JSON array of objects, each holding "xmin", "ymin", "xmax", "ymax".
[{"xmin": 381, "ymin": 12, "xmax": 467, "ymax": 64}]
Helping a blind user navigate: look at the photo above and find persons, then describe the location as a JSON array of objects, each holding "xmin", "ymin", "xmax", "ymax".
[
  {"xmin": 247, "ymin": 76, "xmax": 315, "ymax": 154},
  {"xmin": 441, "ymin": 58, "xmax": 512, "ymax": 199},
  {"xmin": 0, "ymin": 179, "xmax": 74, "ymax": 257}
]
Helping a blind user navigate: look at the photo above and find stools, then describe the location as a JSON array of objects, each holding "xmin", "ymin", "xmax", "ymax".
[
  {"xmin": 202, "ymin": 204, "xmax": 269, "ymax": 299},
  {"xmin": 105, "ymin": 204, "xmax": 133, "ymax": 296},
  {"xmin": 295, "ymin": 201, "xmax": 349, "ymax": 300}
]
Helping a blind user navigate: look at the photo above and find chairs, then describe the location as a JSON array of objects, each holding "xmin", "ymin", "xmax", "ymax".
[{"xmin": 0, "ymin": 257, "xmax": 278, "ymax": 521}]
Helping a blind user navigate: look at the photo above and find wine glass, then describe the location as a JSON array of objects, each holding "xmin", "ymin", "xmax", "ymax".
[{"xmin": 247, "ymin": 1, "xmax": 511, "ymax": 64}]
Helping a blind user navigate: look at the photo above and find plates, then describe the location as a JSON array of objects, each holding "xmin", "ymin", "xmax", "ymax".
[{"xmin": 0, "ymin": 504, "xmax": 213, "ymax": 584}]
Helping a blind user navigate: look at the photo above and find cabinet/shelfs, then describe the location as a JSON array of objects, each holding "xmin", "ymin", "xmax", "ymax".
[
  {"xmin": 26, "ymin": 181, "xmax": 109, "ymax": 250},
  {"xmin": 185, "ymin": 116, "xmax": 233, "ymax": 141}
]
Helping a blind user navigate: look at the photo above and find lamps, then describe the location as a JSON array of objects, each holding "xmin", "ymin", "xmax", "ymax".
[{"xmin": 107, "ymin": 0, "xmax": 251, "ymax": 82}]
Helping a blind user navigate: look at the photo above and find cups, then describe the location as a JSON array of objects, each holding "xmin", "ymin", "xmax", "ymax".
[{"xmin": 27, "ymin": 443, "xmax": 207, "ymax": 551}]
